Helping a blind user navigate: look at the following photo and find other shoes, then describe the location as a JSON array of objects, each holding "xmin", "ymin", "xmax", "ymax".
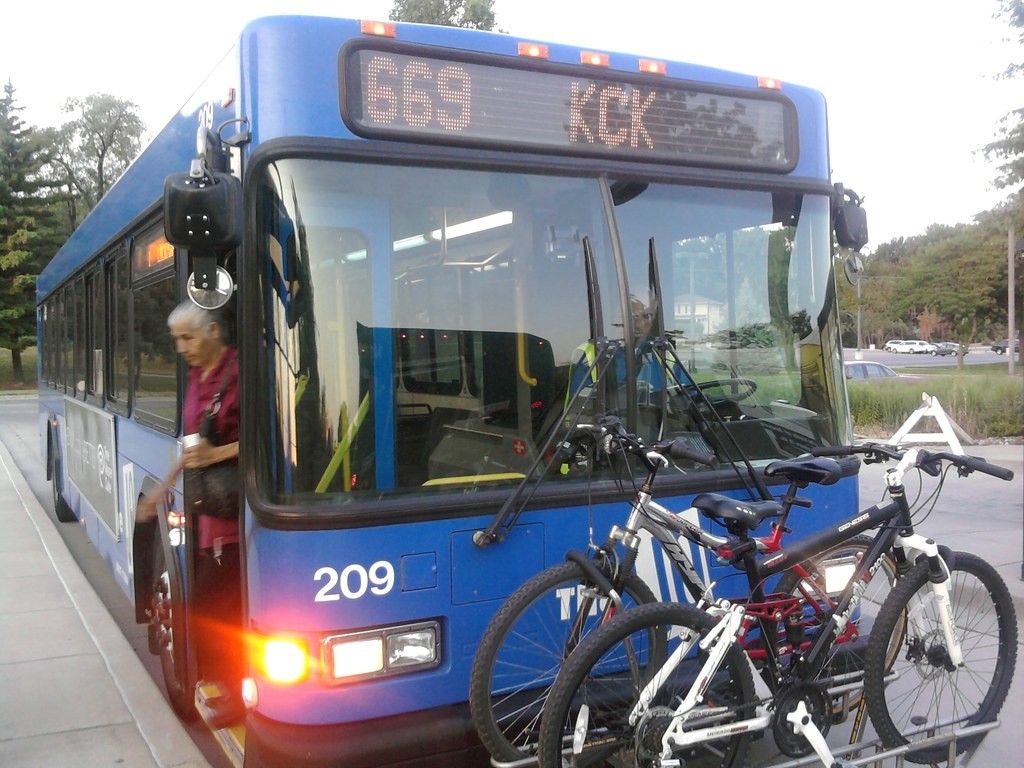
[{"xmin": 204, "ymin": 697, "xmax": 244, "ymax": 730}]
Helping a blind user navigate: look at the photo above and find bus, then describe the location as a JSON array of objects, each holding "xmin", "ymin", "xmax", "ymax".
[{"xmin": 30, "ymin": 16, "xmax": 871, "ymax": 768}]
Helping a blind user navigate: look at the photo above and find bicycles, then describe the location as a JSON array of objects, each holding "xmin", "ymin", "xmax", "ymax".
[
  {"xmin": 469, "ymin": 416, "xmax": 910, "ymax": 768},
  {"xmin": 538, "ymin": 444, "xmax": 1018, "ymax": 768}
]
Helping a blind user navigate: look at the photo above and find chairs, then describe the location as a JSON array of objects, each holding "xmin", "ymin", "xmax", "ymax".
[{"xmin": 352, "ymin": 374, "xmax": 481, "ymax": 490}]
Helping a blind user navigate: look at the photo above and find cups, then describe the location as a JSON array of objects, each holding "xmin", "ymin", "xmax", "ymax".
[{"xmin": 181, "ymin": 433, "xmax": 201, "ymax": 449}]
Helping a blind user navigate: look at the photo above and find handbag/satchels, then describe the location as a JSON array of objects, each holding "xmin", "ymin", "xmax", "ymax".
[{"xmin": 186, "ymin": 355, "xmax": 241, "ymax": 520}]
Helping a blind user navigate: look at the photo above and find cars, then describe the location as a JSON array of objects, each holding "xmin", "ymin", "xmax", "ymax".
[
  {"xmin": 929, "ymin": 342, "xmax": 970, "ymax": 357},
  {"xmin": 844, "ymin": 361, "xmax": 898, "ymax": 380},
  {"xmin": 990, "ymin": 339, "xmax": 1020, "ymax": 355},
  {"xmin": 921, "ymin": 341, "xmax": 939, "ymax": 353},
  {"xmin": 892, "ymin": 340, "xmax": 921, "ymax": 354},
  {"xmin": 884, "ymin": 340, "xmax": 902, "ymax": 352}
]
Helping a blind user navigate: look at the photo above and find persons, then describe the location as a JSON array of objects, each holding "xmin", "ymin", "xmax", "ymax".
[
  {"xmin": 566, "ymin": 285, "xmax": 698, "ymax": 415},
  {"xmin": 167, "ymin": 299, "xmax": 243, "ymax": 733}
]
